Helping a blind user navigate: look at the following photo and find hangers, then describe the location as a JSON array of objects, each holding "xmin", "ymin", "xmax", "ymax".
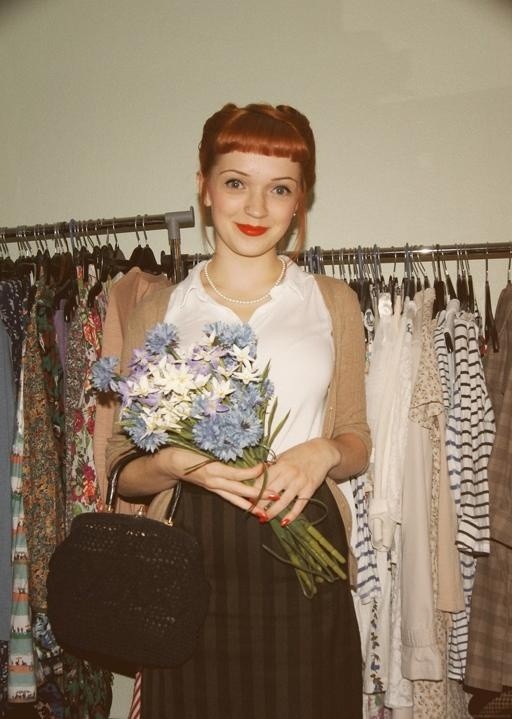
[{"xmin": 0, "ymin": 213, "xmax": 171, "ymax": 320}]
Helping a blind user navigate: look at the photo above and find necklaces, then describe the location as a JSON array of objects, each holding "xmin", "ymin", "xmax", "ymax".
[{"xmin": 203, "ymin": 256, "xmax": 285, "ymax": 305}]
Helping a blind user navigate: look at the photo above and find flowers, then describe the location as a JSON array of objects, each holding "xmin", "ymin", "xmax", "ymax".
[{"xmin": 91, "ymin": 324, "xmax": 348, "ymax": 600}]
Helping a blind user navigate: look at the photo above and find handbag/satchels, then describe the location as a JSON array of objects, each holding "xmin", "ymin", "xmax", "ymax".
[{"xmin": 47, "ymin": 447, "xmax": 209, "ymax": 669}]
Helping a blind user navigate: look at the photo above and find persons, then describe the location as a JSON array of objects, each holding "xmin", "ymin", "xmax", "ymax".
[{"xmin": 104, "ymin": 101, "xmax": 373, "ymax": 718}]
